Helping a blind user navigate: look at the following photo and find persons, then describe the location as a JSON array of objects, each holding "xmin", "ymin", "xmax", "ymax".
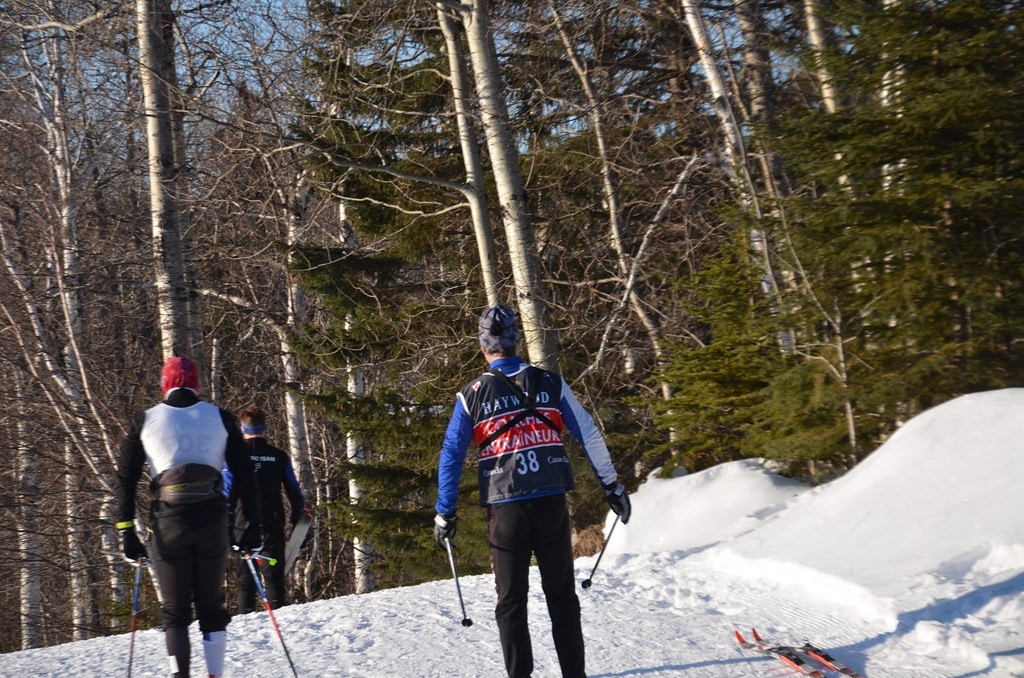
[
  {"xmin": 433, "ymin": 306, "xmax": 632, "ymax": 678},
  {"xmin": 222, "ymin": 407, "xmax": 304, "ymax": 616},
  {"xmin": 115, "ymin": 358, "xmax": 262, "ymax": 678}
]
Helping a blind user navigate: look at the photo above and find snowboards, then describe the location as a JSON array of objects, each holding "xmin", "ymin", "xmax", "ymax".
[{"xmin": 285, "ymin": 514, "xmax": 312, "ymax": 576}]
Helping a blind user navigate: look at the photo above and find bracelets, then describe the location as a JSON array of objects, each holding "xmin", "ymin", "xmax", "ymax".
[{"xmin": 115, "ymin": 521, "xmax": 134, "ymax": 529}]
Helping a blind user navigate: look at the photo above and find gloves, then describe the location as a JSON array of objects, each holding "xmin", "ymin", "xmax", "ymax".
[
  {"xmin": 233, "ymin": 520, "xmax": 265, "ymax": 561},
  {"xmin": 434, "ymin": 513, "xmax": 459, "ymax": 552},
  {"xmin": 604, "ymin": 481, "xmax": 632, "ymax": 524},
  {"xmin": 290, "ymin": 519, "xmax": 314, "ymax": 549},
  {"xmin": 119, "ymin": 526, "xmax": 151, "ymax": 567}
]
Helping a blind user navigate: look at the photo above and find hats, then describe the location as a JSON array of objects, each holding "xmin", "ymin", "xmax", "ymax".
[
  {"xmin": 161, "ymin": 356, "xmax": 200, "ymax": 400},
  {"xmin": 478, "ymin": 305, "xmax": 521, "ymax": 349}
]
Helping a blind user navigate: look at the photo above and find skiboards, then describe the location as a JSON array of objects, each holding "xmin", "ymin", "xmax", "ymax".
[{"xmin": 735, "ymin": 627, "xmax": 865, "ymax": 678}]
